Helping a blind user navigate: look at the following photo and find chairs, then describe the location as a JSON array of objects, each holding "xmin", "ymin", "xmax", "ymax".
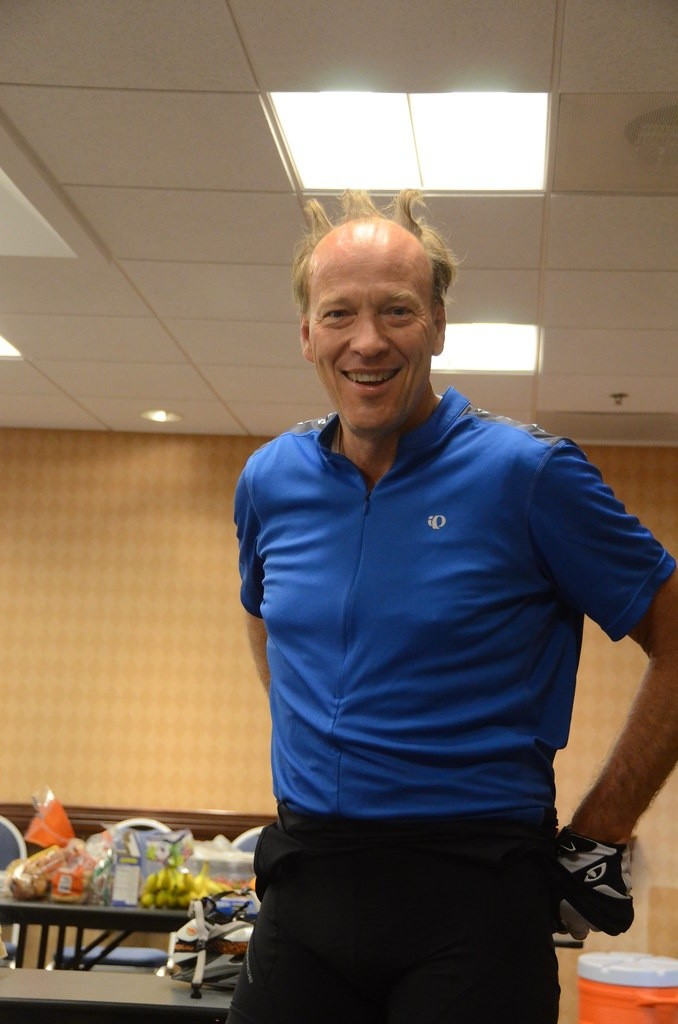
[{"xmin": 0, "ymin": 815, "xmax": 269, "ymax": 970}]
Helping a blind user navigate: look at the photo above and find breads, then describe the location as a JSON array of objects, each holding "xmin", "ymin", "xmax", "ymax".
[{"xmin": 8, "ymin": 845, "xmax": 96, "ymax": 904}]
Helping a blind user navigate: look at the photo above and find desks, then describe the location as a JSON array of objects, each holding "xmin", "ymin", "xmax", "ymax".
[
  {"xmin": 0, "ymin": 967, "xmax": 235, "ymax": 1024},
  {"xmin": 0, "ymin": 894, "xmax": 192, "ymax": 970}
]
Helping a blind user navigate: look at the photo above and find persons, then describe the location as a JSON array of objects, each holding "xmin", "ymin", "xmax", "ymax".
[{"xmin": 233, "ymin": 186, "xmax": 678, "ymax": 1024}]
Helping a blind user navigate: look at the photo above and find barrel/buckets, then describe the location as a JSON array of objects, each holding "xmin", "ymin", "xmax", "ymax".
[{"xmin": 576, "ymin": 951, "xmax": 678, "ymax": 1024}]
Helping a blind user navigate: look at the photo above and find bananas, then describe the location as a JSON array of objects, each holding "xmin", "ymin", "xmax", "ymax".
[{"xmin": 140, "ymin": 861, "xmax": 234, "ymax": 908}]
